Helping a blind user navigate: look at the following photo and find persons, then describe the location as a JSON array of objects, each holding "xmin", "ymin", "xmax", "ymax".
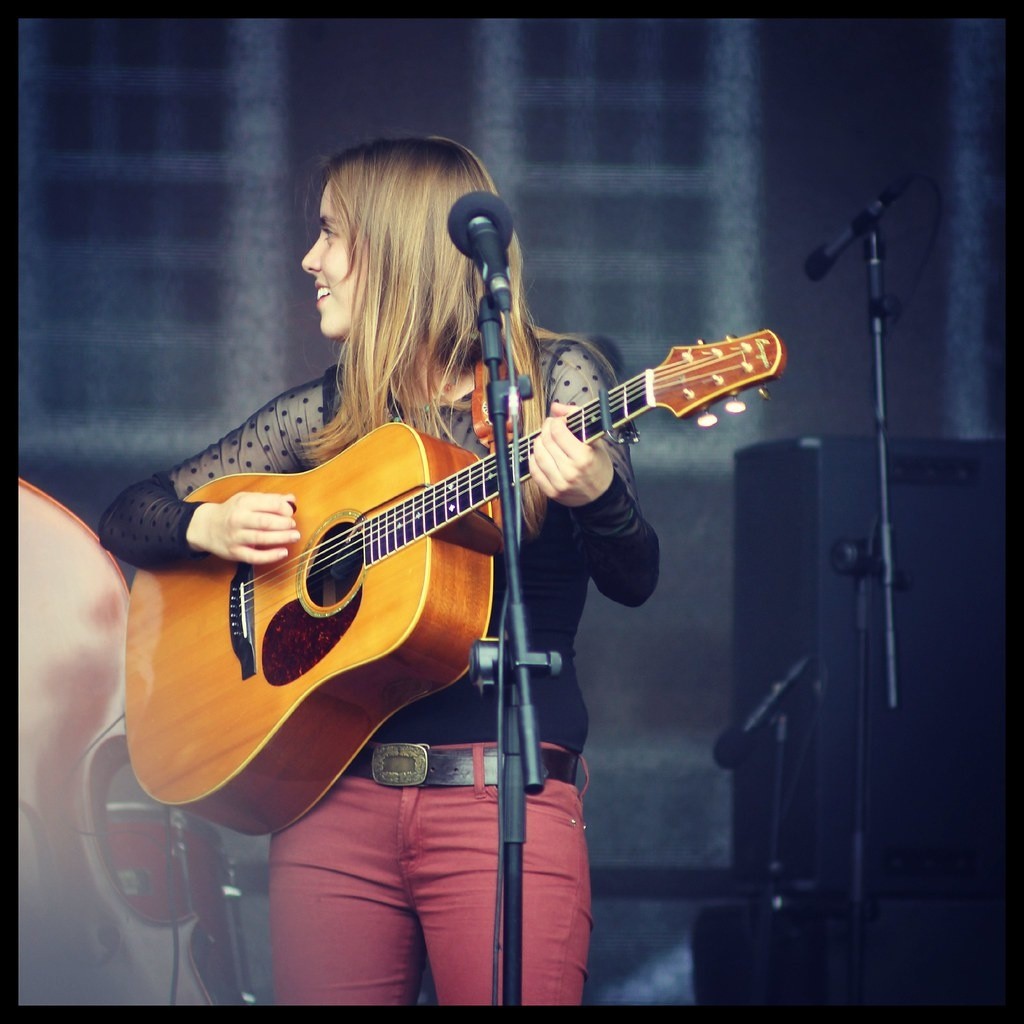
[{"xmin": 95, "ymin": 136, "xmax": 664, "ymax": 1003}]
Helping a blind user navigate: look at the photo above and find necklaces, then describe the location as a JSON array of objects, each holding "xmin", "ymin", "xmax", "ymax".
[{"xmin": 386, "ymin": 368, "xmax": 456, "ymax": 425}]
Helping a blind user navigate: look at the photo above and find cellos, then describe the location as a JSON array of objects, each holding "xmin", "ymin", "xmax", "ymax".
[{"xmin": 18, "ymin": 476, "xmax": 256, "ymax": 1007}]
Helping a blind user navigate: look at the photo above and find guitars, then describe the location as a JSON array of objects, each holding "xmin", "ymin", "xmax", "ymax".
[{"xmin": 126, "ymin": 328, "xmax": 788, "ymax": 837}]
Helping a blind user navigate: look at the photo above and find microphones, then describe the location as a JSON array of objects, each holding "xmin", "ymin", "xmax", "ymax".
[
  {"xmin": 806, "ymin": 174, "xmax": 919, "ymax": 280},
  {"xmin": 447, "ymin": 191, "xmax": 513, "ymax": 312},
  {"xmin": 715, "ymin": 654, "xmax": 813, "ymax": 769}
]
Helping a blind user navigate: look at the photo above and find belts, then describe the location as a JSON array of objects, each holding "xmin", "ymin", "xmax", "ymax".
[{"xmin": 343, "ymin": 743, "xmax": 579, "ymax": 787}]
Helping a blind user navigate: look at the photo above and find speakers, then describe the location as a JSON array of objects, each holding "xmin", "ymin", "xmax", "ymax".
[
  {"xmin": 727, "ymin": 430, "xmax": 1005, "ymax": 887},
  {"xmin": 693, "ymin": 888, "xmax": 1007, "ymax": 1010}
]
